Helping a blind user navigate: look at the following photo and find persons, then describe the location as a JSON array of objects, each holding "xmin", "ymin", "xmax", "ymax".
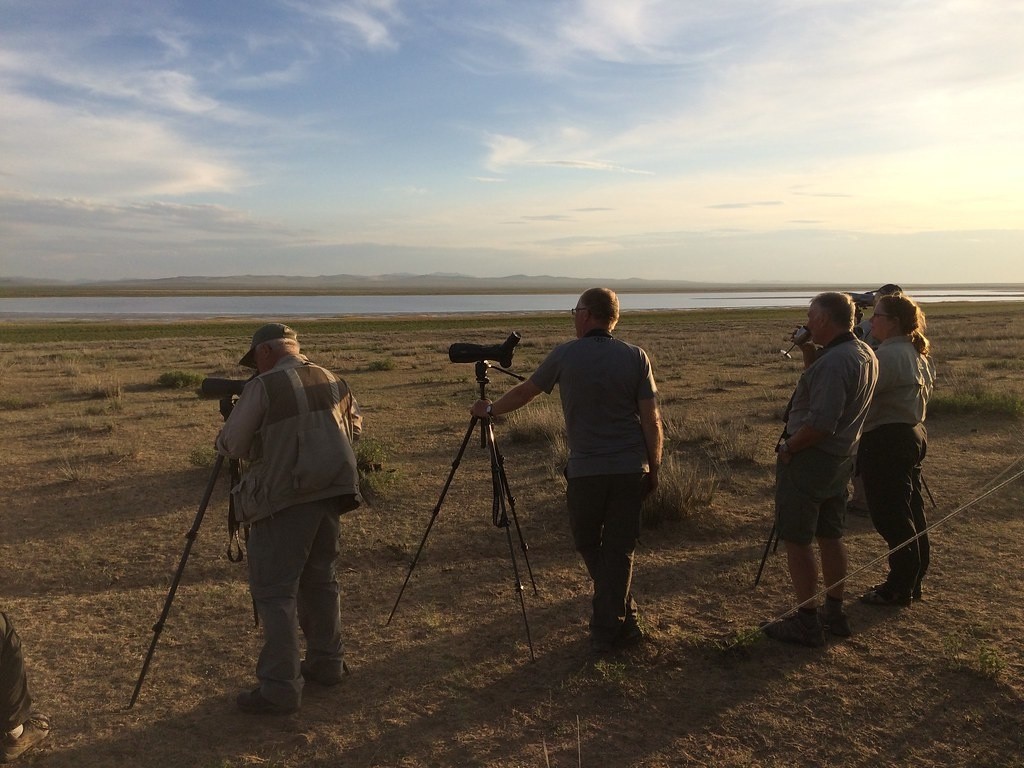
[
  {"xmin": 211, "ymin": 323, "xmax": 365, "ymax": 717},
  {"xmin": 469, "ymin": 287, "xmax": 665, "ymax": 654},
  {"xmin": 844, "ymin": 283, "xmax": 909, "ymax": 519},
  {"xmin": 854, "ymin": 293, "xmax": 932, "ymax": 609},
  {"xmin": 1, "ymin": 610, "xmax": 51, "ymax": 762},
  {"xmin": 755, "ymin": 290, "xmax": 880, "ymax": 642}
]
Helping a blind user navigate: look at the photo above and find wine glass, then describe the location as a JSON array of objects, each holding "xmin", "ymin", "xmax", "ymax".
[{"xmin": 780, "ymin": 325, "xmax": 811, "ymax": 359}]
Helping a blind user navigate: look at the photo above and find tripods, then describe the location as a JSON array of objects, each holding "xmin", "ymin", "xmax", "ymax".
[
  {"xmin": 384, "ymin": 360, "xmax": 539, "ymax": 662},
  {"xmin": 128, "ymin": 398, "xmax": 352, "ymax": 711}
]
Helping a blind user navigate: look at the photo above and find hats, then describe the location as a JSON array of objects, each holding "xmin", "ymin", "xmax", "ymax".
[
  {"xmin": 872, "ymin": 284, "xmax": 902, "ymax": 295},
  {"xmin": 238, "ymin": 323, "xmax": 296, "ymax": 369}
]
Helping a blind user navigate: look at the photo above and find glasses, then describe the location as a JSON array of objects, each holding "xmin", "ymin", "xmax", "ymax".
[
  {"xmin": 571, "ymin": 308, "xmax": 592, "ymax": 317},
  {"xmin": 873, "ymin": 311, "xmax": 893, "ymax": 319}
]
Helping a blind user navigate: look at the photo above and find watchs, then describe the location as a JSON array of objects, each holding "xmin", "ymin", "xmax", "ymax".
[
  {"xmin": 485, "ymin": 404, "xmax": 494, "ymax": 416},
  {"xmin": 782, "ymin": 442, "xmax": 793, "ymax": 454}
]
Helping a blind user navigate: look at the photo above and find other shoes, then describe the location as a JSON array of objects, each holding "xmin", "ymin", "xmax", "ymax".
[
  {"xmin": 237, "ymin": 688, "xmax": 301, "ymax": 715},
  {"xmin": 870, "ymin": 581, "xmax": 922, "ymax": 600},
  {"xmin": 589, "ymin": 632, "xmax": 613, "ymax": 650},
  {"xmin": 819, "ymin": 604, "xmax": 850, "ymax": 636},
  {"xmin": 300, "ymin": 659, "xmax": 342, "ymax": 686},
  {"xmin": 760, "ymin": 611, "xmax": 830, "ymax": 646},
  {"xmin": 858, "ymin": 583, "xmax": 913, "ymax": 606},
  {"xmin": 0, "ymin": 714, "xmax": 50, "ymax": 761},
  {"xmin": 621, "ymin": 623, "xmax": 644, "ymax": 642}
]
